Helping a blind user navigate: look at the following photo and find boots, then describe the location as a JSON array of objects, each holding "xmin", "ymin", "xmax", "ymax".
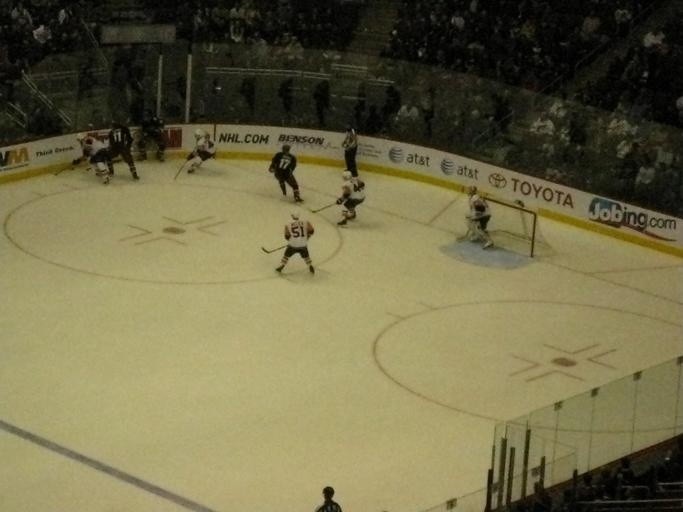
[
  {"xmin": 275, "ymin": 265, "xmax": 284, "ymax": 272},
  {"xmin": 157, "ymin": 150, "xmax": 164, "ymax": 161},
  {"xmin": 294, "ymin": 194, "xmax": 304, "ymax": 201},
  {"xmin": 137, "ymin": 152, "xmax": 147, "ymax": 161},
  {"xmin": 337, "ymin": 216, "xmax": 346, "ymax": 224}
]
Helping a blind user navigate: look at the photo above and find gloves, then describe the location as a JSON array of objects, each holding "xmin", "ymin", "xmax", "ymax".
[{"xmin": 336, "ymin": 199, "xmax": 342, "ymax": 204}]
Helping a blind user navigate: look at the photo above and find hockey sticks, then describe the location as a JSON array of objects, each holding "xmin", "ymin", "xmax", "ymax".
[
  {"xmin": 262, "ymin": 245, "xmax": 287, "ymax": 253},
  {"xmin": 456, "ymin": 194, "xmax": 488, "ymax": 240},
  {"xmin": 308, "ymin": 203, "xmax": 336, "ymax": 213},
  {"xmin": 51, "ymin": 159, "xmax": 81, "ymax": 176}
]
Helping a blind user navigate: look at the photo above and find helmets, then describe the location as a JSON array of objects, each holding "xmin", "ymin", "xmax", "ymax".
[
  {"xmin": 465, "ymin": 185, "xmax": 477, "ymax": 194},
  {"xmin": 282, "ymin": 144, "xmax": 291, "ymax": 151},
  {"xmin": 290, "ymin": 210, "xmax": 300, "ymax": 220},
  {"xmin": 342, "ymin": 171, "xmax": 351, "ymax": 180}
]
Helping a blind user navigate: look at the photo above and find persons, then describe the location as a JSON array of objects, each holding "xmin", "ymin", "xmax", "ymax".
[
  {"xmin": 135, "ymin": 106, "xmax": 166, "ymax": 162},
  {"xmin": 380, "ymin": 0, "xmax": 683, "ymax": 220},
  {"xmin": 106, "ymin": 115, "xmax": 139, "ymax": 181},
  {"xmin": 466, "ymin": 185, "xmax": 494, "ymax": 249},
  {"xmin": 530, "ymin": 432, "xmax": 682, "ymax": 511},
  {"xmin": 274, "ymin": 208, "xmax": 315, "ymax": 275},
  {"xmin": 185, "ymin": 129, "xmax": 216, "ymax": 174},
  {"xmin": 313, "ymin": 486, "xmax": 342, "ymax": 511},
  {"xmin": 335, "ymin": 171, "xmax": 365, "ymax": 225},
  {"xmin": 340, "ymin": 122, "xmax": 358, "ymax": 178},
  {"xmin": 75, "ymin": 132, "xmax": 111, "ymax": 186},
  {"xmin": 0, "ymin": 1, "xmax": 381, "ymax": 146},
  {"xmin": 271, "ymin": 144, "xmax": 304, "ymax": 203}
]
